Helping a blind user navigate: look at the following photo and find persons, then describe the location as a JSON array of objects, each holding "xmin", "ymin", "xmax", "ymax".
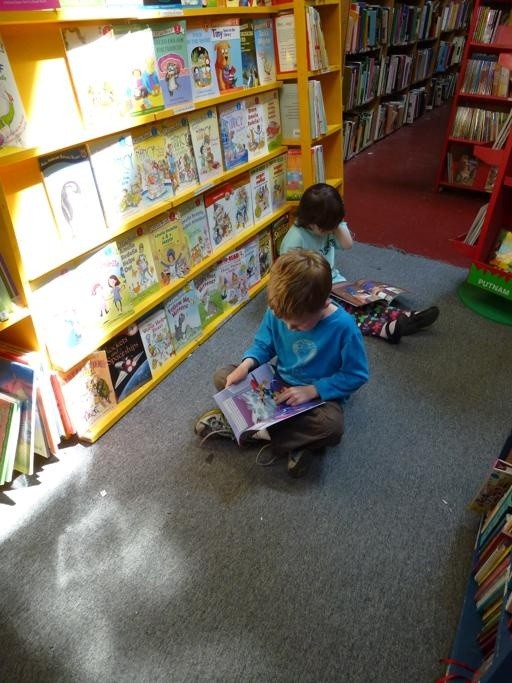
[
  {"xmin": 194, "ymin": 245, "xmax": 371, "ymax": 480},
  {"xmin": 277, "ymin": 180, "xmax": 440, "ymax": 346}
]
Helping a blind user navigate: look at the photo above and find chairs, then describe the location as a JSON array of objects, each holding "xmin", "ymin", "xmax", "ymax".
[
  {"xmin": 285, "ymin": 448, "xmax": 313, "ymax": 477},
  {"xmin": 194, "ymin": 408, "xmax": 235, "ymax": 442}
]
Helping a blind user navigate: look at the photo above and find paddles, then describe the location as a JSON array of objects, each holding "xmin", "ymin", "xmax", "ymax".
[{"xmin": 1, "ymin": 241, "xmax": 512, "ymax": 681}]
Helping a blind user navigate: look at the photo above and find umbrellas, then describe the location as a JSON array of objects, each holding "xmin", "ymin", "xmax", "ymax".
[
  {"xmin": 382, "ymin": 315, "xmax": 406, "ymax": 343},
  {"xmin": 401, "ymin": 305, "xmax": 440, "ymax": 335}
]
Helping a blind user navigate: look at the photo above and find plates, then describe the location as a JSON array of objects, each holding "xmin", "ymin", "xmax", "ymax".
[
  {"xmin": 2, "ymin": 0, "xmax": 285, "ymax": 444},
  {"xmin": 272, "ymin": 0, "xmax": 349, "ymax": 229},
  {"xmin": 435, "ymin": 0, "xmax": 510, "ymax": 195},
  {"xmin": 343, "ymin": 0, "xmax": 458, "ymax": 159}
]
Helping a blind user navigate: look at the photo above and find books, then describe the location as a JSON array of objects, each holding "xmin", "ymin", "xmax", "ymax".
[
  {"xmin": 1, "ymin": 350, "xmax": 68, "ymax": 491},
  {"xmin": 160, "ymin": 211, "xmax": 289, "ymax": 352},
  {"xmin": 446, "ymin": 2, "xmax": 512, "ymax": 190},
  {"xmin": 469, "ymin": 442, "xmax": 511, "ymax": 681},
  {"xmin": 487, "ymin": 229, "xmax": 511, "ymax": 274},
  {"xmin": 99, "ymin": 308, "xmax": 176, "ymax": 405},
  {"xmin": 2, "ymin": 35, "xmax": 34, "ymax": 150},
  {"xmin": 37, "ymin": 89, "xmax": 290, "ymax": 245},
  {"xmin": 277, "ymin": 3, "xmax": 329, "ymax": 196},
  {"xmin": 37, "ymin": 207, "xmax": 191, "ymax": 354},
  {"xmin": 324, "ymin": 277, "xmax": 410, "ymax": 309},
  {"xmin": 61, "ymin": 347, "xmax": 117, "ymax": 427},
  {"xmin": 210, "ymin": 361, "xmax": 330, "ymax": 448},
  {"xmin": 1, "ymin": 259, "xmax": 21, "ymax": 320},
  {"xmin": 0, "ymin": 1, "xmax": 284, "ymax": 12},
  {"xmin": 462, "ymin": 203, "xmax": 488, "ymax": 248},
  {"xmin": 59, "ymin": 20, "xmax": 280, "ymax": 125},
  {"xmin": 341, "ymin": 0, "xmax": 461, "ymax": 157}
]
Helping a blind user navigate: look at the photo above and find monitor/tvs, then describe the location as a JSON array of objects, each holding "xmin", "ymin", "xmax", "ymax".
[
  {"xmin": 462, "ymin": 203, "xmax": 488, "ymax": 248},
  {"xmin": 446, "ymin": 2, "xmax": 512, "ymax": 190},
  {"xmin": 1, "ymin": 350, "xmax": 68, "ymax": 491},
  {"xmin": 487, "ymin": 229, "xmax": 511, "ymax": 274},
  {"xmin": 469, "ymin": 442, "xmax": 511, "ymax": 681},
  {"xmin": 341, "ymin": 0, "xmax": 461, "ymax": 157},
  {"xmin": 277, "ymin": 3, "xmax": 329, "ymax": 196},
  {"xmin": 210, "ymin": 361, "xmax": 330, "ymax": 448},
  {"xmin": 324, "ymin": 277, "xmax": 410, "ymax": 309}
]
